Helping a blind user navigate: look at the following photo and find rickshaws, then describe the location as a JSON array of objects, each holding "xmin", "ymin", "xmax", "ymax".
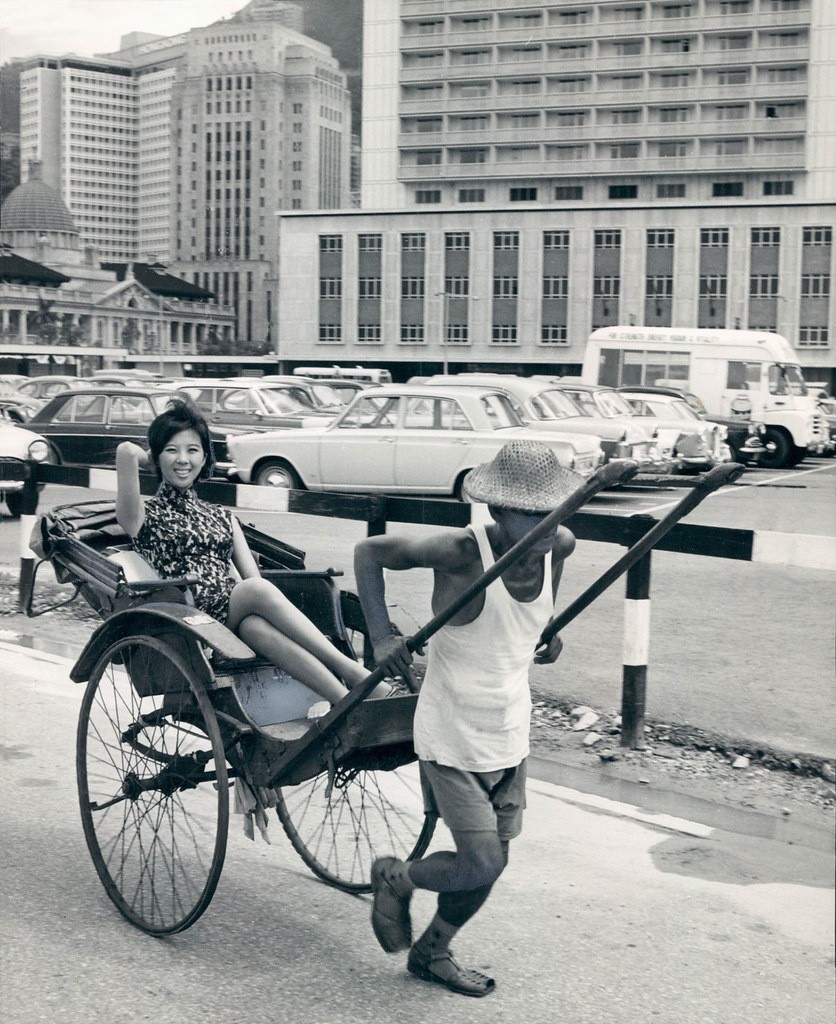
[{"xmin": 26, "ymin": 460, "xmax": 746, "ymax": 938}]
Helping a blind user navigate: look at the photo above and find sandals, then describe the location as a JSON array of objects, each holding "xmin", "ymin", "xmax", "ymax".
[
  {"xmin": 407, "ymin": 943, "xmax": 496, "ymax": 998},
  {"xmin": 371, "ymin": 857, "xmax": 412, "ymax": 955}
]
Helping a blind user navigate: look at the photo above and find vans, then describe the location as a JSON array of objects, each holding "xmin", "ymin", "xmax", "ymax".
[{"xmin": 582, "ymin": 325, "xmax": 831, "ymax": 469}]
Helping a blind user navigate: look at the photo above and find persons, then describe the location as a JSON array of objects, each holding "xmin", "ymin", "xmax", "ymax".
[
  {"xmin": 114, "ymin": 404, "xmax": 407, "ymax": 702},
  {"xmin": 352, "ymin": 443, "xmax": 584, "ymax": 997}
]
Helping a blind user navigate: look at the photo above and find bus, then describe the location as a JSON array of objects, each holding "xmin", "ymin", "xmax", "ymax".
[{"xmin": 292, "ymin": 365, "xmax": 391, "ymax": 405}]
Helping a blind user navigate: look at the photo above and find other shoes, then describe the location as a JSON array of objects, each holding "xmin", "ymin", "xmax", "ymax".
[{"xmin": 386, "ymin": 685, "xmax": 410, "ymax": 698}]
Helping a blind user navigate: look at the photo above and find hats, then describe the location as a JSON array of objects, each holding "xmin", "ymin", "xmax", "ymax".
[{"xmin": 464, "ymin": 440, "xmax": 586, "ymax": 513}]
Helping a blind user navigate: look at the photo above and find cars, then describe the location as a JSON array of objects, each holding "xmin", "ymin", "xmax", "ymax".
[{"xmin": 0, "ymin": 369, "xmax": 733, "ymax": 517}]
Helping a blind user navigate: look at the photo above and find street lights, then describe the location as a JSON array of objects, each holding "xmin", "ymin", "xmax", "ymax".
[
  {"xmin": 143, "ymin": 294, "xmax": 179, "ymax": 374},
  {"xmin": 434, "ymin": 291, "xmax": 480, "ymax": 375}
]
